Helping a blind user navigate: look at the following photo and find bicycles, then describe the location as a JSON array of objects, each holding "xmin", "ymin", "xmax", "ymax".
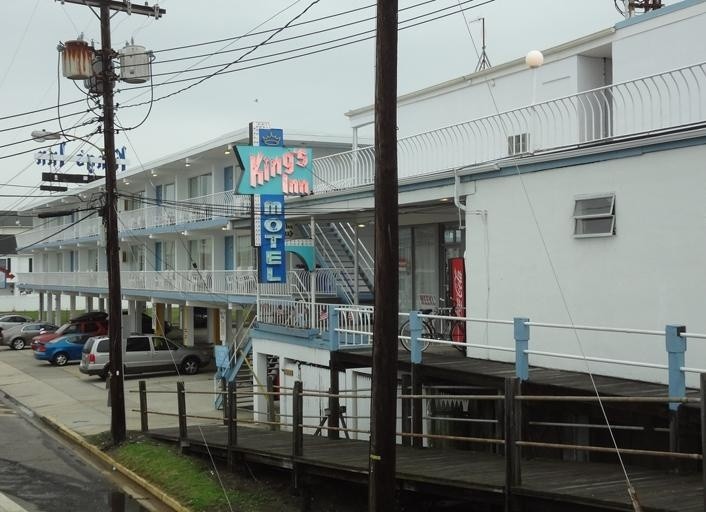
[{"xmin": 400, "ymin": 298, "xmax": 466, "ymax": 353}]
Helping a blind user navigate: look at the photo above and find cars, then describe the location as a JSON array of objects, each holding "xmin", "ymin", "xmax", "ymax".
[{"xmin": 0, "ymin": 310, "xmax": 210, "ymax": 380}]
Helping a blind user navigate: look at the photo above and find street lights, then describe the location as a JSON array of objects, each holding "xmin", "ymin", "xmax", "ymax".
[
  {"xmin": 31, "ymin": 128, "xmax": 126, "ymax": 445},
  {"xmin": 525, "ymin": 48, "xmax": 544, "ymax": 158}
]
